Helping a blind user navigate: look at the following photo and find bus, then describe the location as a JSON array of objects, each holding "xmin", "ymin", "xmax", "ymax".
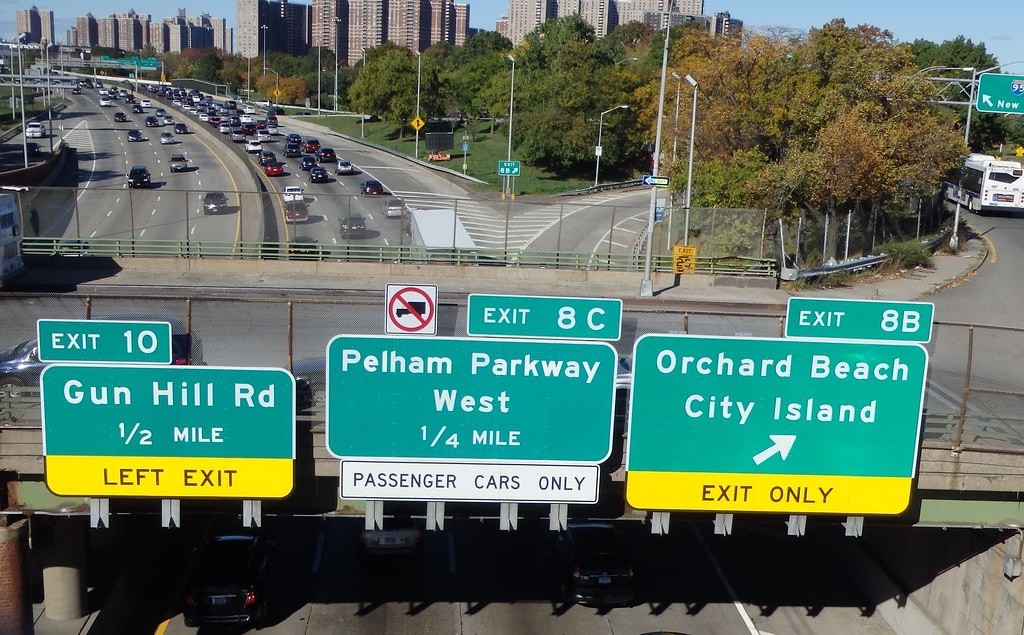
[
  {"xmin": 941, "ymin": 153, "xmax": 1024, "ymax": 213},
  {"xmin": 0, "ymin": 192, "xmax": 28, "ymax": 286}
]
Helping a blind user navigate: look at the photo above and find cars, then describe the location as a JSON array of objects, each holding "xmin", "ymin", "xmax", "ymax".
[
  {"xmin": 282, "ymin": 200, "xmax": 316, "ymax": 223},
  {"xmin": 160, "ymin": 131, "xmax": 175, "ymax": 144},
  {"xmin": 23, "ymin": 142, "xmax": 39, "ymax": 157},
  {"xmin": 163, "ymin": 115, "xmax": 176, "ymax": 125},
  {"xmin": 346, "ymin": 516, "xmax": 426, "ymax": 555},
  {"xmin": 280, "ymin": 186, "xmax": 306, "ymax": 203},
  {"xmin": 143, "ymin": 116, "xmax": 160, "ymax": 127},
  {"xmin": 289, "ymin": 236, "xmax": 320, "ymax": 260},
  {"xmin": 174, "ymin": 123, "xmax": 190, "ymax": 135},
  {"xmin": 127, "ymin": 128, "xmax": 142, "ymax": 143},
  {"xmin": 360, "ymin": 180, "xmax": 385, "ymax": 197},
  {"xmin": 167, "ymin": 154, "xmax": 191, "ymax": 173},
  {"xmin": 183, "ymin": 532, "xmax": 274, "ymax": 629},
  {"xmin": 309, "ymin": 166, "xmax": 328, "ymax": 184},
  {"xmin": 114, "ymin": 111, "xmax": 127, "ymax": 122},
  {"xmin": 337, "ymin": 214, "xmax": 368, "ymax": 238},
  {"xmin": 26, "ymin": 122, "xmax": 47, "ymax": 139},
  {"xmin": 288, "ymin": 356, "xmax": 328, "ymax": 412},
  {"xmin": 66, "ymin": 77, "xmax": 338, "ymax": 177},
  {"xmin": 553, "ymin": 523, "xmax": 642, "ymax": 605},
  {"xmin": 383, "ymin": 198, "xmax": 408, "ymax": 219},
  {"xmin": 202, "ymin": 191, "xmax": 229, "ymax": 214},
  {"xmin": 335, "ymin": 159, "xmax": 355, "ymax": 176}
]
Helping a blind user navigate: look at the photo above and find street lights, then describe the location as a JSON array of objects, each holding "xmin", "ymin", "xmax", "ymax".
[
  {"xmin": 323, "ymin": 70, "xmax": 337, "ymax": 114},
  {"xmin": 61, "ymin": 35, "xmax": 67, "ymax": 99},
  {"xmin": 673, "ymin": 70, "xmax": 681, "ymax": 160},
  {"xmin": 9, "ymin": 36, "xmax": 18, "ymax": 122},
  {"xmin": 46, "ymin": 42, "xmax": 55, "ymax": 155},
  {"xmin": 316, "ymin": 39, "xmax": 322, "ymax": 115},
  {"xmin": 592, "ymin": 104, "xmax": 629, "ymax": 187},
  {"xmin": 360, "ymin": 47, "xmax": 367, "ymax": 137},
  {"xmin": 506, "ymin": 54, "xmax": 516, "ymax": 195},
  {"xmin": 414, "ymin": 49, "xmax": 422, "ymax": 157},
  {"xmin": 260, "ymin": 24, "xmax": 270, "ymax": 76},
  {"xmin": 17, "ymin": 32, "xmax": 30, "ymax": 168},
  {"xmin": 683, "ymin": 72, "xmax": 697, "ymax": 252},
  {"xmin": 263, "ymin": 67, "xmax": 279, "ymax": 105},
  {"xmin": 39, "ymin": 36, "xmax": 47, "ymax": 109},
  {"xmin": 332, "ymin": 18, "xmax": 341, "ymax": 115}
]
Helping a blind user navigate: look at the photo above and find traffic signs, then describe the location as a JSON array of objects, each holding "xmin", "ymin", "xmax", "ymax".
[
  {"xmin": 621, "ymin": 338, "xmax": 923, "ymax": 521},
  {"xmin": 646, "ymin": 177, "xmax": 672, "ymax": 190},
  {"xmin": 498, "ymin": 160, "xmax": 521, "ymax": 177},
  {"xmin": 975, "ymin": 73, "xmax": 1024, "ymax": 115}
]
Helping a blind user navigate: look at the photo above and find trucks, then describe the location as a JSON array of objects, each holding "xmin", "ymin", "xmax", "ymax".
[{"xmin": 408, "ymin": 210, "xmax": 479, "ymax": 266}]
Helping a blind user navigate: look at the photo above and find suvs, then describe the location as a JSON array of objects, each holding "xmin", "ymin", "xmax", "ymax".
[
  {"xmin": 127, "ymin": 165, "xmax": 153, "ymax": 189},
  {"xmin": 0, "ymin": 315, "xmax": 207, "ymax": 387}
]
